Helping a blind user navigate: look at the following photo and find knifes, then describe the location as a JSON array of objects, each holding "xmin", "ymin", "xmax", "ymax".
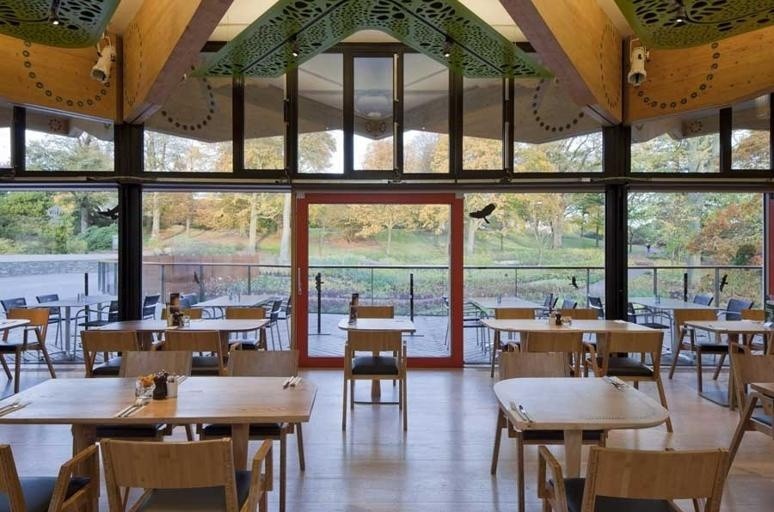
[{"xmin": 519, "ymin": 405, "xmax": 535, "ymax": 424}]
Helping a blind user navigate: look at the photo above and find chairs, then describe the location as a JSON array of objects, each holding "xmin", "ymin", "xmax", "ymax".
[{"xmin": 342, "ymin": 305, "xmax": 407, "ymax": 435}]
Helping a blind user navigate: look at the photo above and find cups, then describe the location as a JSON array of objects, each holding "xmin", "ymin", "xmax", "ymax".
[{"xmin": 183, "ymin": 315, "xmax": 191, "ymax": 326}]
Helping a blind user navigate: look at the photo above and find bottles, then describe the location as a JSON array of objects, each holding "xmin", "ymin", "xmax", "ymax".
[
  {"xmin": 548, "ymin": 311, "xmax": 562, "ymax": 327},
  {"xmin": 153, "ymin": 371, "xmax": 177, "ymax": 399},
  {"xmin": 172, "ymin": 314, "xmax": 182, "ymax": 326}
]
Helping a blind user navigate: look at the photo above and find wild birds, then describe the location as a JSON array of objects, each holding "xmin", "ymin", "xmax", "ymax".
[
  {"xmin": 469, "ymin": 204, "xmax": 495, "ymax": 223},
  {"xmin": 98, "ymin": 205, "xmax": 119, "ymax": 220}
]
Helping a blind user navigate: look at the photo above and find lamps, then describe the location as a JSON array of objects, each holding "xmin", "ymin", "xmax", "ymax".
[
  {"xmin": 627, "ymin": 39, "xmax": 650, "ymax": 86},
  {"xmin": 291, "ymin": 48, "xmax": 299, "ymax": 56},
  {"xmin": 443, "ymin": 50, "xmax": 451, "ymax": 59},
  {"xmin": 52, "ymin": 17, "xmax": 59, "ymax": 25},
  {"xmin": 676, "ymin": 15, "xmax": 683, "ymax": 23},
  {"xmin": 91, "ymin": 36, "xmax": 115, "ymax": 82}
]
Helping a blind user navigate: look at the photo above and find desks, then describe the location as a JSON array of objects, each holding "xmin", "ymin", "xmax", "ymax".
[{"xmin": 338, "ymin": 317, "xmax": 415, "ymax": 335}]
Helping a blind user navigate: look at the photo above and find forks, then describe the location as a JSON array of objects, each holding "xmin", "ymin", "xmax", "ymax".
[
  {"xmin": 115, "ymin": 396, "xmax": 146, "ymax": 418},
  {"xmin": 510, "ymin": 399, "xmax": 525, "ymax": 424},
  {"xmin": 0, "ymin": 398, "xmax": 31, "ymax": 418}
]
